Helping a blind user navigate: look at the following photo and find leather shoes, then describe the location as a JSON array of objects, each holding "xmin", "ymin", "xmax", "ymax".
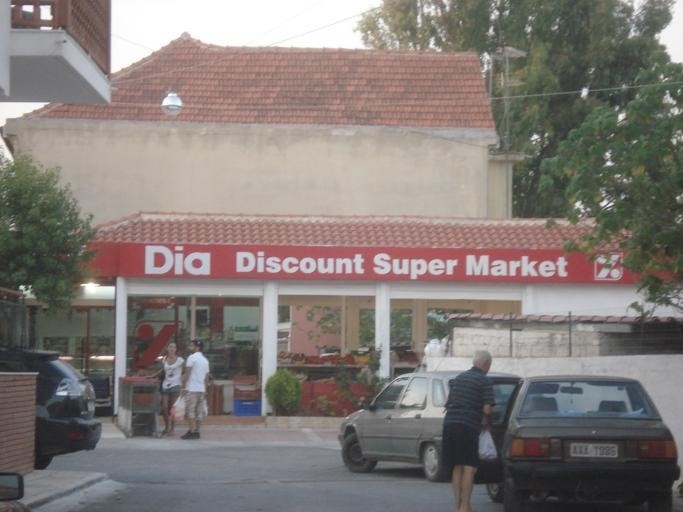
[{"xmin": 181, "ymin": 430, "xmax": 200, "ymax": 439}]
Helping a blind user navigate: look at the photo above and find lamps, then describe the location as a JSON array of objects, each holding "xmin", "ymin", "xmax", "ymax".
[{"xmin": 161, "ymin": 92, "xmax": 182, "ymax": 116}]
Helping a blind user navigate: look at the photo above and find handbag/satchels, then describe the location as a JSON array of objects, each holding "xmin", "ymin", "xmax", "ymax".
[{"xmin": 169, "ymin": 397, "xmax": 185, "ymax": 421}]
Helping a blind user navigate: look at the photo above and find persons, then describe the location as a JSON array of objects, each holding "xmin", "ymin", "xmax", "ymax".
[
  {"xmin": 146, "ymin": 341, "xmax": 186, "ymax": 434},
  {"xmin": 180, "ymin": 340, "xmax": 210, "ymax": 439},
  {"xmin": 442, "ymin": 350, "xmax": 496, "ymax": 512}
]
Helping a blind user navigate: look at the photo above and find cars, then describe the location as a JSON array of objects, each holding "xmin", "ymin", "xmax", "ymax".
[
  {"xmin": 473, "ymin": 373, "xmax": 681, "ymax": 511},
  {"xmin": 336, "ymin": 370, "xmax": 466, "ymax": 483},
  {"xmin": 1, "ymin": 344, "xmax": 103, "ymax": 471}
]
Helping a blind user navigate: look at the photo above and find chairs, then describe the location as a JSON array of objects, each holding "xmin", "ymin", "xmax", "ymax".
[{"xmin": 91, "ymin": 372, "xmax": 112, "ymax": 409}]
